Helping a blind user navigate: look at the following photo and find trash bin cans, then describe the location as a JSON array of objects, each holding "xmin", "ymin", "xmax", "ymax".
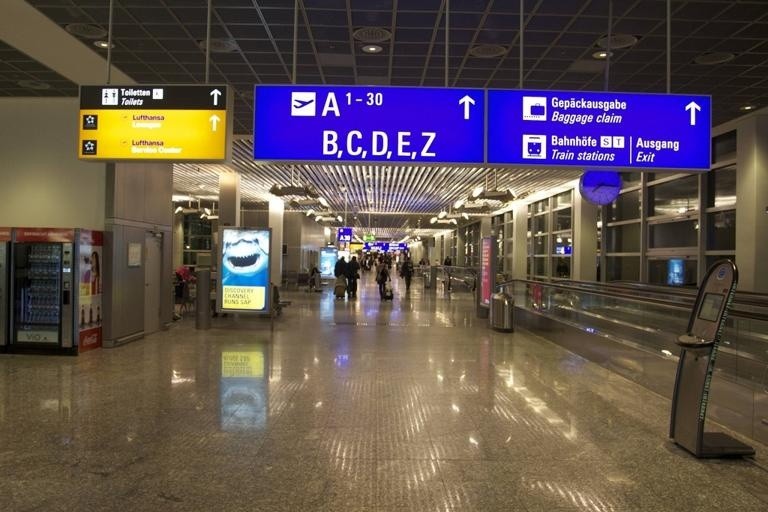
[
  {"xmin": 423, "ymin": 272, "xmax": 431, "ymax": 289},
  {"xmin": 489, "ymin": 292, "xmax": 513, "ymax": 333}
]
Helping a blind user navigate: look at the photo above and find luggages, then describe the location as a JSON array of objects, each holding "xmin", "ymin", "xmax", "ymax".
[
  {"xmin": 383, "ymin": 280, "xmax": 393, "ymax": 302},
  {"xmin": 335, "ymin": 286, "xmax": 345, "ymax": 299}
]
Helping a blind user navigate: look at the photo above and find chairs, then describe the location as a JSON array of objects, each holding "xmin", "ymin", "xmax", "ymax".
[
  {"xmin": 281, "ymin": 270, "xmax": 311, "ymax": 292},
  {"xmin": 172, "ymin": 282, "xmax": 195, "ymax": 315}
]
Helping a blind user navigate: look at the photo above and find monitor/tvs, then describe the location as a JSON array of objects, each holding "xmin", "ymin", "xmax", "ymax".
[{"xmin": 699, "ymin": 293, "xmax": 724, "ymax": 321}]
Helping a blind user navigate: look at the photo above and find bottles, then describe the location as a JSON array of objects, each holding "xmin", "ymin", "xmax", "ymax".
[
  {"xmin": 24, "ymin": 244, "xmax": 61, "ymax": 325},
  {"xmin": 81, "ymin": 303, "xmax": 100, "ymax": 329}
]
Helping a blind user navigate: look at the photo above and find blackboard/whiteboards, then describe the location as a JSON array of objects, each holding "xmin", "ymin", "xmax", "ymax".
[{"xmin": 127, "ymin": 242, "xmax": 142, "ymax": 267}]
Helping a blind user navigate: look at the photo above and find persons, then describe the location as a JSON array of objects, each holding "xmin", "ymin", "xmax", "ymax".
[
  {"xmin": 419, "ymin": 258, "xmax": 430, "ymax": 268},
  {"xmin": 435, "ymin": 259, "xmax": 440, "ymax": 265},
  {"xmin": 444, "ymin": 256, "xmax": 452, "ymax": 266},
  {"xmin": 81, "ymin": 252, "xmax": 100, "ymax": 295},
  {"xmin": 174, "ymin": 266, "xmax": 195, "ymax": 318},
  {"xmin": 335, "ymin": 250, "xmax": 414, "ymax": 299}
]
